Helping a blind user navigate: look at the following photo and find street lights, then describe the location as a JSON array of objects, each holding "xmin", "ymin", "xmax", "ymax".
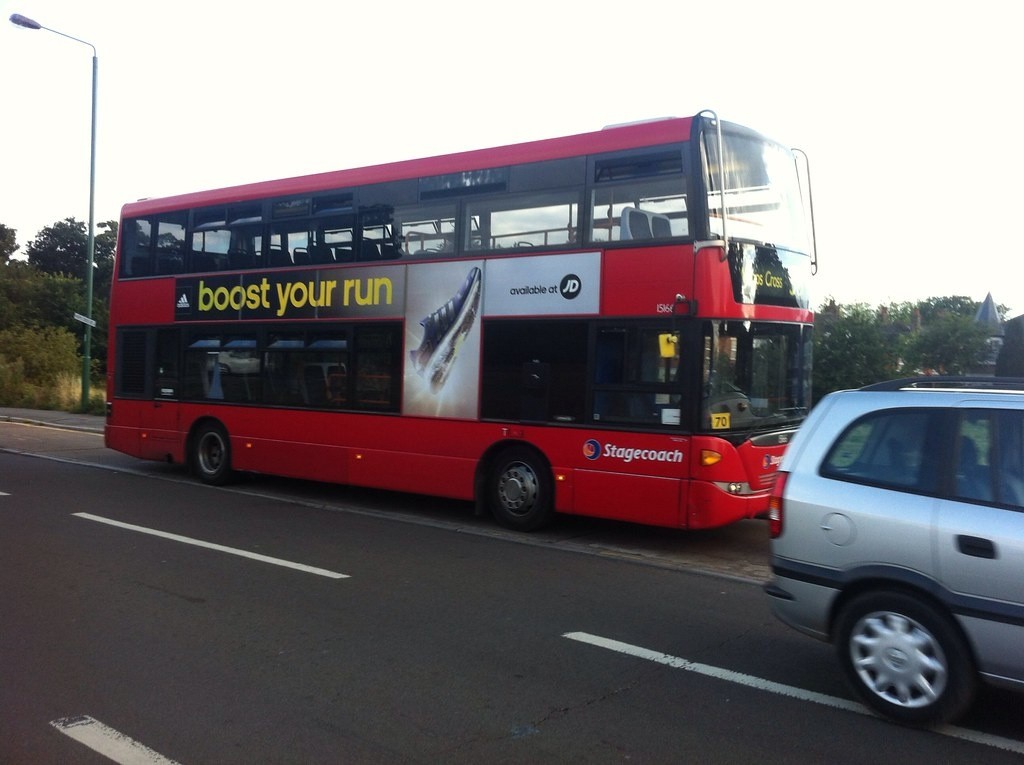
[{"xmin": 10, "ymin": 13, "xmax": 97, "ymax": 412}]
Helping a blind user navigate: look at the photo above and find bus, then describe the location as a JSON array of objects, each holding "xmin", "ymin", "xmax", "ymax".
[{"xmin": 104, "ymin": 114, "xmax": 816, "ymax": 537}]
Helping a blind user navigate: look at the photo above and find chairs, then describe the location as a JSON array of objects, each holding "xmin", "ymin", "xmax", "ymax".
[
  {"xmin": 133, "ymin": 237, "xmax": 535, "ymax": 275},
  {"xmin": 947, "ymin": 434, "xmax": 1013, "ymax": 506},
  {"xmin": 186, "ymin": 359, "xmax": 402, "ymax": 413}
]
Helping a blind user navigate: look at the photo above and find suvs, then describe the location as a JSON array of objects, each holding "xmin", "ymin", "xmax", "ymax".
[{"xmin": 765, "ymin": 376, "xmax": 1024, "ymax": 725}]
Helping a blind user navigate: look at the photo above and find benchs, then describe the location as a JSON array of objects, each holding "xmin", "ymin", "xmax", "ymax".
[
  {"xmin": 876, "ymin": 465, "xmax": 935, "ymax": 491},
  {"xmin": 619, "ymin": 206, "xmax": 673, "ymax": 240}
]
